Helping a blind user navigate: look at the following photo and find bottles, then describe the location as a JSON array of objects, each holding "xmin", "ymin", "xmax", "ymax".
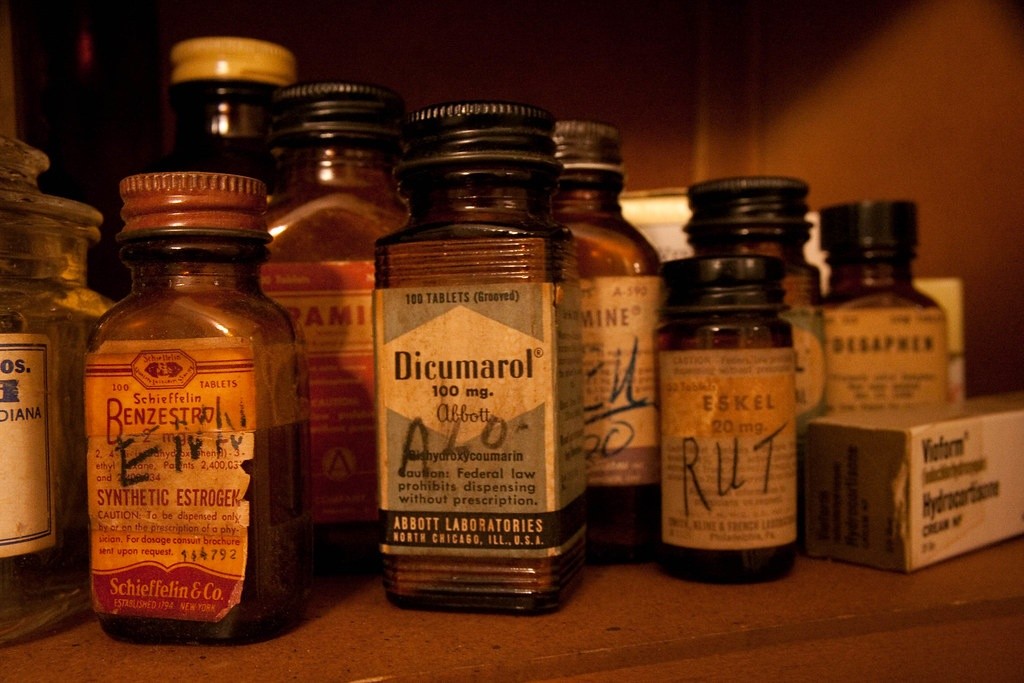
[
  {"xmin": 370, "ymin": 100, "xmax": 583, "ymax": 614},
  {"xmin": 660, "ymin": 258, "xmax": 798, "ymax": 578},
  {"xmin": 82, "ymin": 171, "xmax": 313, "ymax": 648},
  {"xmin": 550, "ymin": 118, "xmax": 666, "ymax": 565},
  {"xmin": 817, "ymin": 193, "xmax": 948, "ymax": 413},
  {"xmin": 255, "ymin": 82, "xmax": 410, "ymax": 575},
  {"xmin": 171, "ymin": 34, "xmax": 298, "ymax": 191},
  {"xmin": 683, "ymin": 174, "xmax": 824, "ymax": 421},
  {"xmin": 0, "ymin": 136, "xmax": 118, "ymax": 645}
]
